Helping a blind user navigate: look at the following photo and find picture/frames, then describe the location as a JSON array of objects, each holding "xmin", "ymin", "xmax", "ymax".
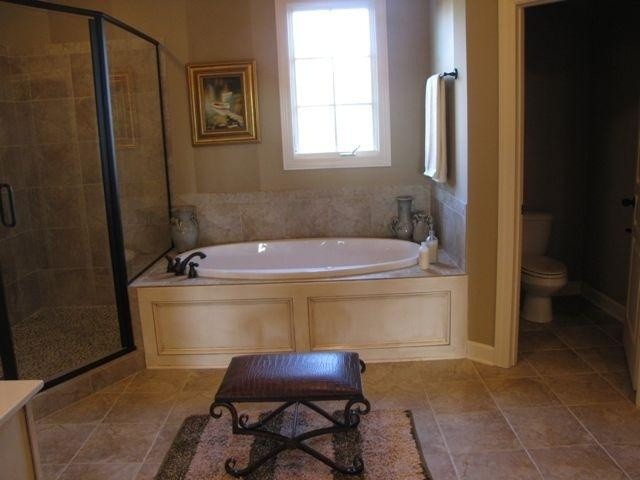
[{"xmin": 187, "ymin": 58, "xmax": 262, "ymax": 147}]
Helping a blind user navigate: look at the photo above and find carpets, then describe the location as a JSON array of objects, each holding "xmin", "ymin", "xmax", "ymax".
[{"xmin": 152, "ymin": 409, "xmax": 434, "ymax": 479}]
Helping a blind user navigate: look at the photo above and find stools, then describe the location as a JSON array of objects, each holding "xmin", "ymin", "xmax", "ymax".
[{"xmin": 210, "ymin": 352, "xmax": 372, "ymax": 476}]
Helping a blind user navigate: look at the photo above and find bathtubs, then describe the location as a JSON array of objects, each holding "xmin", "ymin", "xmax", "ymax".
[{"xmin": 170, "ymin": 236, "xmax": 423, "ymax": 280}]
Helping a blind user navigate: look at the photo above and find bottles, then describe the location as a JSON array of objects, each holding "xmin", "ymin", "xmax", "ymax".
[{"xmin": 420, "ymin": 230, "xmax": 438, "ymax": 269}]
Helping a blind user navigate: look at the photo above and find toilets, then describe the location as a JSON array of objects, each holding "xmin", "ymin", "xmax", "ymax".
[{"xmin": 520, "ymin": 210, "xmax": 569, "ymax": 324}]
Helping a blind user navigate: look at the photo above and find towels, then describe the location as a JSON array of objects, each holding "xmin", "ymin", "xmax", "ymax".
[{"xmin": 423, "ymin": 74, "xmax": 448, "ymax": 184}]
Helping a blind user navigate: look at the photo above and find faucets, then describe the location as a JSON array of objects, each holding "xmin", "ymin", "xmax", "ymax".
[{"xmin": 172, "ymin": 252, "xmax": 207, "ymax": 276}]
